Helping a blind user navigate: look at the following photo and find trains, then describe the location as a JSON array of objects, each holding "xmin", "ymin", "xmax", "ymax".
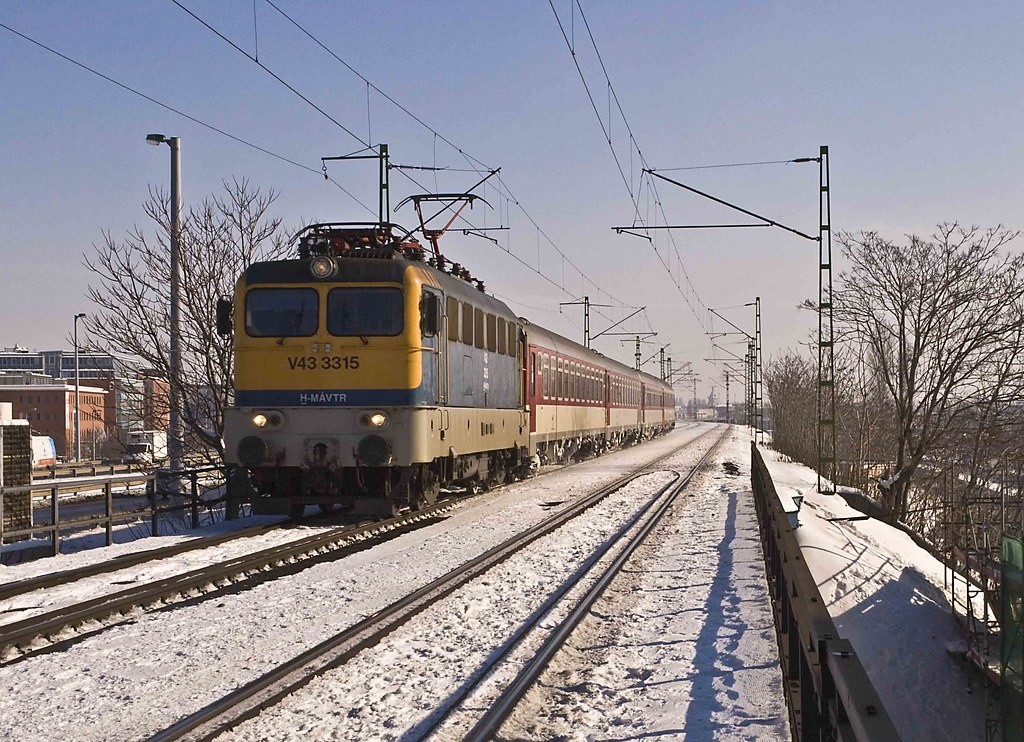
[{"xmin": 215, "ymin": 145, "xmax": 678, "ymax": 519}]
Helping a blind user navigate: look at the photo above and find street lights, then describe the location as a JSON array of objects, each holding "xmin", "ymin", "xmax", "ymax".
[
  {"xmin": 145, "ymin": 133, "xmax": 183, "ymax": 494},
  {"xmin": 74, "ymin": 313, "xmax": 87, "ymax": 463}
]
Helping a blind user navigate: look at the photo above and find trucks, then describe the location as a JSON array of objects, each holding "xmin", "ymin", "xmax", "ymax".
[{"xmin": 122, "ymin": 431, "xmax": 168, "ymax": 465}]
